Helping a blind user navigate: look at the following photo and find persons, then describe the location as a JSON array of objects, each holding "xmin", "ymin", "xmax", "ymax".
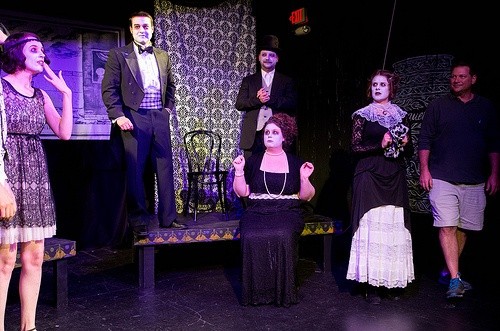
[
  {"xmin": 237, "ymin": 36, "xmax": 296, "ymax": 210},
  {"xmin": 346, "ymin": 70, "xmax": 416, "ymax": 305},
  {"xmin": 0, "ymin": 30, "xmax": 10, "ymax": 45},
  {"xmin": 102, "ymin": 12, "xmax": 191, "ymax": 236},
  {"xmin": 232, "ymin": 113, "xmax": 315, "ymax": 305},
  {"xmin": 418, "ymin": 64, "xmax": 499, "ymax": 294},
  {"xmin": 0, "ymin": 33, "xmax": 72, "ymax": 331}
]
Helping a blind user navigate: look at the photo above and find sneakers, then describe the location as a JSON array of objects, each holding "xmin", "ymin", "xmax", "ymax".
[{"xmin": 446, "ymin": 272, "xmax": 472, "ymax": 297}]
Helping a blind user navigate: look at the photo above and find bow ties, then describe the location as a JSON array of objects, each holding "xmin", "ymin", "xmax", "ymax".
[{"xmin": 133, "ymin": 41, "xmax": 153, "ymax": 54}]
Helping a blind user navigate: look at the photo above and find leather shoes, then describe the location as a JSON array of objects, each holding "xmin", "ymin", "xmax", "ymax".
[
  {"xmin": 159, "ymin": 219, "xmax": 189, "ymax": 229},
  {"xmin": 129, "ymin": 224, "xmax": 149, "ymax": 237}
]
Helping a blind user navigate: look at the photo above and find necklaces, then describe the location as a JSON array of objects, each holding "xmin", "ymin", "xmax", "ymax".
[
  {"xmin": 264, "ymin": 151, "xmax": 287, "ymax": 198},
  {"xmin": 370, "ymin": 104, "xmax": 389, "ymax": 115}
]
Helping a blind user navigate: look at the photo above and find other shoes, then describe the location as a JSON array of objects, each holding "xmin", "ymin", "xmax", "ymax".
[{"xmin": 364, "ymin": 286, "xmax": 401, "ymax": 304}]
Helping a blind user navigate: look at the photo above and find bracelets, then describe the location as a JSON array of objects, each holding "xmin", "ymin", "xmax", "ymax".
[{"xmin": 235, "ymin": 171, "xmax": 244, "ymax": 176}]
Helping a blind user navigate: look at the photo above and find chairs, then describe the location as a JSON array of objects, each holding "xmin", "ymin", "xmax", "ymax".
[{"xmin": 182, "ymin": 130, "xmax": 230, "ymax": 221}]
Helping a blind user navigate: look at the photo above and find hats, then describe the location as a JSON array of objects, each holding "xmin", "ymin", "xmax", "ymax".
[{"xmin": 256, "ymin": 35, "xmax": 281, "ymax": 56}]
